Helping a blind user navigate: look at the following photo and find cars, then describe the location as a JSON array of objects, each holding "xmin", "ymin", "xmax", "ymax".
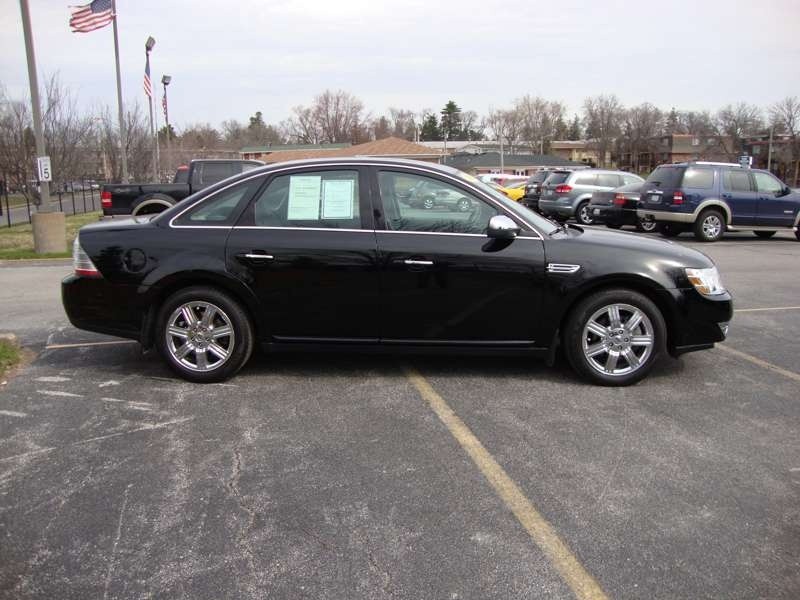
[{"xmin": 60, "ymin": 156, "xmax": 733, "ymax": 386}]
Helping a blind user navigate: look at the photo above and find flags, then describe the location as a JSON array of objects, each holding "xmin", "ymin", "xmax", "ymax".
[
  {"xmin": 162, "ymin": 94, "xmax": 168, "ymax": 122},
  {"xmin": 67, "ymin": 0, "xmax": 117, "ymax": 34},
  {"xmin": 143, "ymin": 55, "xmax": 152, "ymax": 97}
]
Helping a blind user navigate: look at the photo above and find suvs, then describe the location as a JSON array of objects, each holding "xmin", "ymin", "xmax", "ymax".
[
  {"xmin": 404, "ymin": 165, "xmax": 644, "ymax": 229},
  {"xmin": 637, "ymin": 155, "xmax": 800, "ymax": 243}
]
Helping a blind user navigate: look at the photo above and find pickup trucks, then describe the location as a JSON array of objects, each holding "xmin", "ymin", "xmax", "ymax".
[{"xmin": 98, "ymin": 159, "xmax": 271, "ymax": 218}]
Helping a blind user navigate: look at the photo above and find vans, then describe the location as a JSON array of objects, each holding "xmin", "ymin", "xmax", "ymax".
[{"xmin": 71, "ymin": 179, "xmax": 100, "ymax": 191}]
